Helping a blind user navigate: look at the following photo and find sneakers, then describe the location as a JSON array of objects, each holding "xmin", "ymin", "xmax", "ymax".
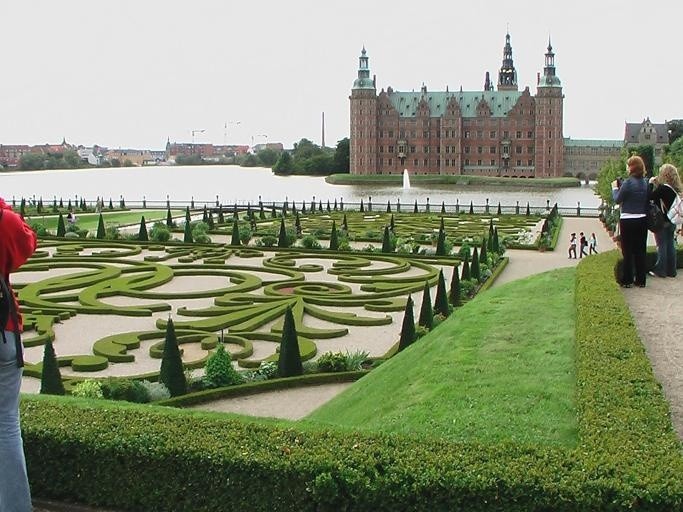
[{"xmin": 618, "ymin": 270, "xmax": 677, "ymax": 288}]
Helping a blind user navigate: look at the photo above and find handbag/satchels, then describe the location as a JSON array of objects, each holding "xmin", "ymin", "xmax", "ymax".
[
  {"xmin": 584, "ymin": 241, "xmax": 588, "ymax": 246},
  {"xmin": 647, "ymin": 201, "xmax": 665, "ymax": 233}
]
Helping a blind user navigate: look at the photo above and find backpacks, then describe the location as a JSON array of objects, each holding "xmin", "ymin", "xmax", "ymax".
[
  {"xmin": 659, "ymin": 183, "xmax": 683, "ymax": 224},
  {"xmin": 0, "ymin": 274, "xmax": 21, "ymax": 343}
]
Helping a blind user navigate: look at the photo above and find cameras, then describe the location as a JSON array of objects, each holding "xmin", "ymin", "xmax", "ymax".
[
  {"xmin": 653, "ymin": 178, "xmax": 656, "ymax": 182},
  {"xmin": 616, "ymin": 177, "xmax": 623, "ymax": 185}
]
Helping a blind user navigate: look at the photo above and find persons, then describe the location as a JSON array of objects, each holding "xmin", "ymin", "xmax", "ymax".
[
  {"xmin": 578, "ymin": 232, "xmax": 588, "ymax": 259},
  {"xmin": 609, "ymin": 155, "xmax": 653, "ymax": 289},
  {"xmin": 567, "ymin": 232, "xmax": 576, "ymax": 259},
  {"xmin": 588, "ymin": 233, "xmax": 598, "ymax": 255},
  {"xmin": 645, "ymin": 163, "xmax": 682, "ymax": 280},
  {"xmin": 0, "ymin": 195, "xmax": 37, "ymax": 512}
]
